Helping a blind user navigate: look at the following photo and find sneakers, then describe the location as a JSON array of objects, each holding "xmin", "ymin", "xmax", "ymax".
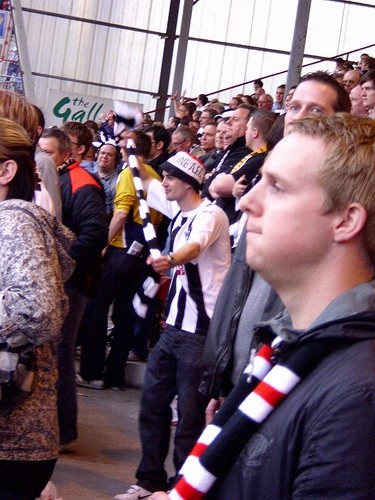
[{"xmin": 113, "ymin": 483, "xmax": 152, "ymax": 500}]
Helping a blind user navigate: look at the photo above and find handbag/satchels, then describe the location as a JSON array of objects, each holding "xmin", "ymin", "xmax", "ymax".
[{"xmin": 1, "ymin": 340, "xmax": 39, "ymax": 397}]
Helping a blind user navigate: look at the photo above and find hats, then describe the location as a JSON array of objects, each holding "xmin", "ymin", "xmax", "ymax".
[
  {"xmin": 159, "ymin": 150, "xmax": 206, "ymax": 193},
  {"xmin": 214, "ymin": 110, "xmax": 235, "ymax": 121}
]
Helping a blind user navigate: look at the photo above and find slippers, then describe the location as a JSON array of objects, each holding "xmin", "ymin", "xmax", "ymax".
[{"xmin": 58, "ymin": 448, "xmax": 76, "ymax": 454}]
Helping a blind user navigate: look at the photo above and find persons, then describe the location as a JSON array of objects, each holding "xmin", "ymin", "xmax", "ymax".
[{"xmin": 1, "ymin": 53, "xmax": 375, "ymax": 500}]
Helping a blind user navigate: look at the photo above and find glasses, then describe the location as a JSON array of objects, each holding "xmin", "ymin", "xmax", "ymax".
[
  {"xmin": 257, "ymin": 101, "xmax": 271, "ymax": 105},
  {"xmin": 342, "ymin": 79, "xmax": 358, "ymax": 85}
]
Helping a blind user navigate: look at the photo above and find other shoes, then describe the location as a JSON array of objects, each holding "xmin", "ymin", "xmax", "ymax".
[
  {"xmin": 74, "ymin": 372, "xmax": 122, "ymax": 390},
  {"xmin": 74, "ymin": 327, "xmax": 158, "ymax": 362}
]
